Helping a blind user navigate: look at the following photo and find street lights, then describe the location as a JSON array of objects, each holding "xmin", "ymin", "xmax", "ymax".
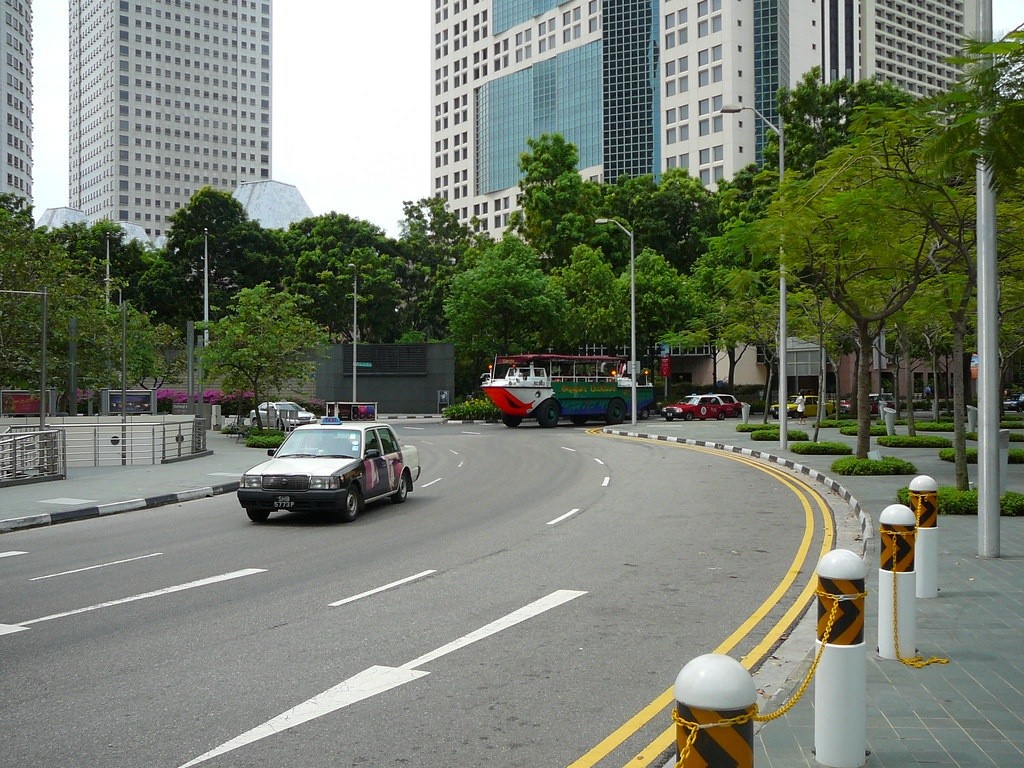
[
  {"xmin": 594, "ymin": 218, "xmax": 638, "ymax": 425},
  {"xmin": 347, "ymin": 263, "xmax": 358, "ymax": 402},
  {"xmin": 718, "ymin": 103, "xmax": 788, "ymax": 451}
]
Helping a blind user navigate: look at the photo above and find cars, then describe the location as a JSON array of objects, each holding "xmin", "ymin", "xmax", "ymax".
[
  {"xmin": 661, "ymin": 393, "xmax": 751, "ymax": 422},
  {"xmin": 236, "ymin": 415, "xmax": 422, "ymax": 524},
  {"xmin": 1003, "ymin": 394, "xmax": 1024, "ymax": 413},
  {"xmin": 769, "ymin": 395, "xmax": 834, "ymax": 419},
  {"xmin": 834, "ymin": 392, "xmax": 896, "ymax": 415},
  {"xmin": 249, "ymin": 402, "xmax": 317, "ymax": 431}
]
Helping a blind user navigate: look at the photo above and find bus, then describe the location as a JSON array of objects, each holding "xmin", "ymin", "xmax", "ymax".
[{"xmin": 481, "ymin": 353, "xmax": 655, "ymax": 430}]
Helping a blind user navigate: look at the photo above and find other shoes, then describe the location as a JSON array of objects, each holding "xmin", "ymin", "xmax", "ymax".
[
  {"xmin": 798, "ymin": 421, "xmax": 801, "ymax": 425},
  {"xmin": 803, "ymin": 420, "xmax": 806, "ymax": 424}
]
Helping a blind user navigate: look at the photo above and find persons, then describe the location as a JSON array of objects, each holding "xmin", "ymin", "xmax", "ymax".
[
  {"xmin": 926, "ymin": 385, "xmax": 932, "ymax": 400},
  {"xmin": 796, "ymin": 389, "xmax": 806, "ymax": 425}
]
